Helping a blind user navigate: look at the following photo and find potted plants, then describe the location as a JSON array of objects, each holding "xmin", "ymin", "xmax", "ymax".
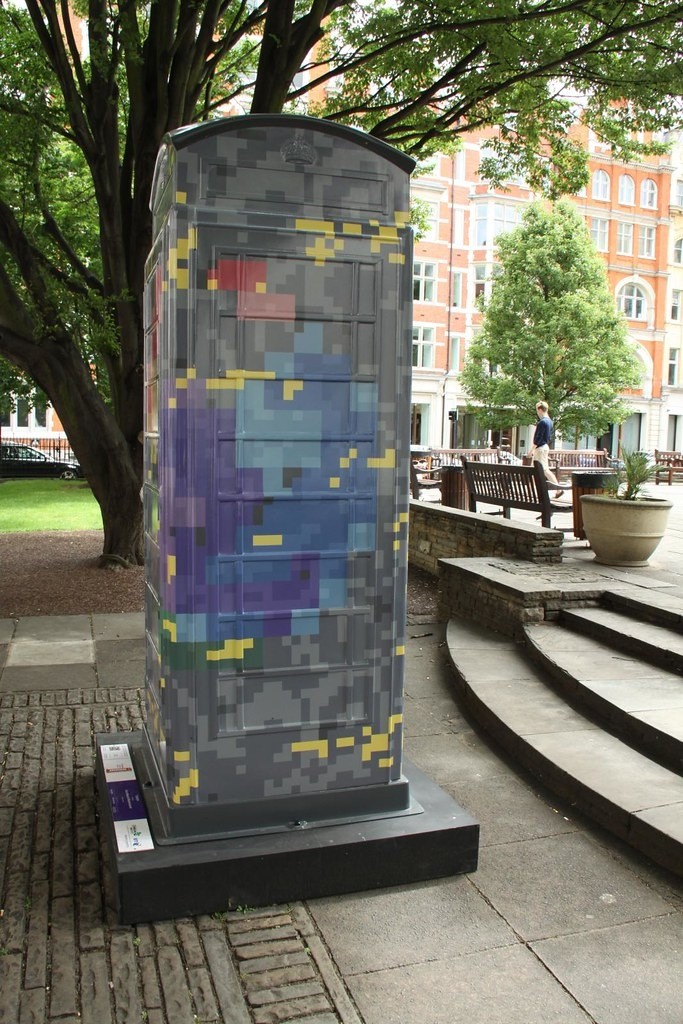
[{"xmin": 580, "ymin": 440, "xmax": 674, "ymax": 567}]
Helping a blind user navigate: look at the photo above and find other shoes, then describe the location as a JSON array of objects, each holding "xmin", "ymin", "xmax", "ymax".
[{"xmin": 553, "ymin": 490, "xmax": 565, "ymax": 499}]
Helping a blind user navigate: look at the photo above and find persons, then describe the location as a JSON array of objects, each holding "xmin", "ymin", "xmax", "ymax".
[{"xmin": 526, "ymin": 401, "xmax": 565, "ymax": 500}]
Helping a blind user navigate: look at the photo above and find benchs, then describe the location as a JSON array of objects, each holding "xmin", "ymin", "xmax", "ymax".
[
  {"xmin": 410, "ymin": 450, "xmax": 446, "ymax": 503},
  {"xmin": 547, "ymin": 448, "xmax": 621, "ymax": 483},
  {"xmin": 429, "ymin": 447, "xmax": 506, "ymax": 488},
  {"xmin": 460, "ymin": 454, "xmax": 574, "ymax": 529},
  {"xmin": 655, "ymin": 449, "xmax": 683, "ymax": 485}
]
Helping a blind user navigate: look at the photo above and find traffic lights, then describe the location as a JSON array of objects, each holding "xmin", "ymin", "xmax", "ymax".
[{"xmin": 449, "ymin": 411, "xmax": 457, "ymax": 421}]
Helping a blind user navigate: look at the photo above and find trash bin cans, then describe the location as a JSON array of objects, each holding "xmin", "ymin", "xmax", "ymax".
[
  {"xmin": 440, "ymin": 465, "xmax": 469, "ymax": 511},
  {"xmin": 571, "ymin": 470, "xmax": 618, "ymax": 540}
]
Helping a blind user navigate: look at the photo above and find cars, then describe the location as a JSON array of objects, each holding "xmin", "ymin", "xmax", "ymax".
[{"xmin": 0, "ymin": 440, "xmax": 85, "ymax": 481}]
